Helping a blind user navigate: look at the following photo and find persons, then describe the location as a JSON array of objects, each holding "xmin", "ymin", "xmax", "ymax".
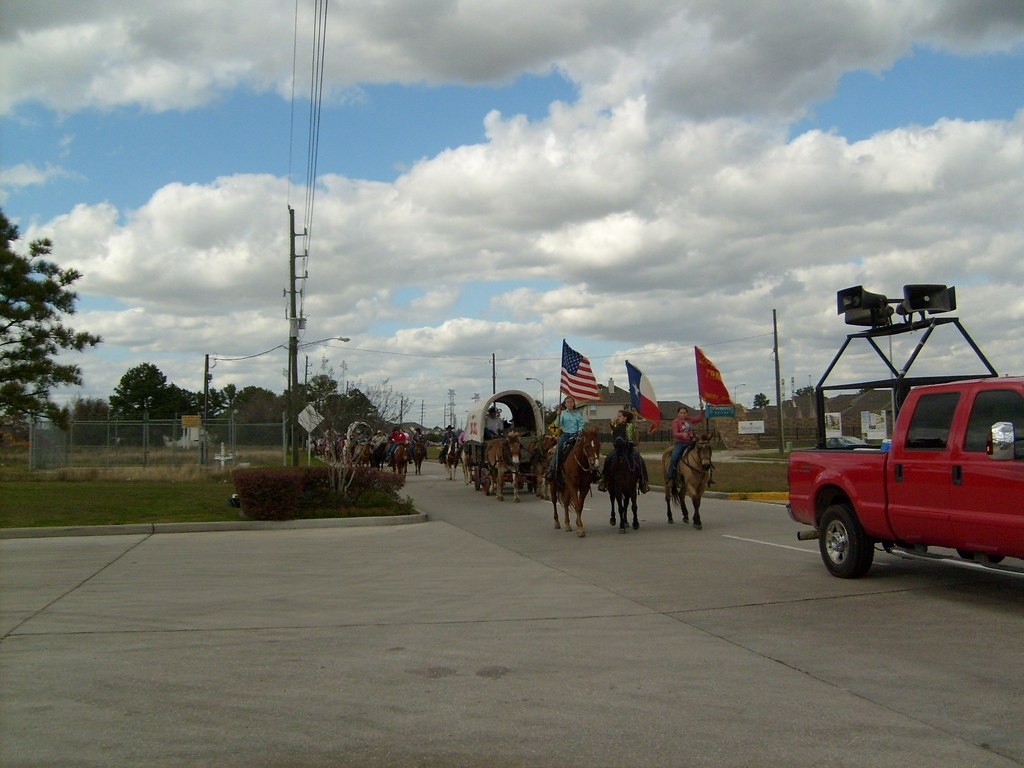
[
  {"xmin": 441, "ymin": 425, "xmax": 458, "ymax": 453},
  {"xmin": 485, "ymin": 407, "xmax": 504, "ymax": 435},
  {"xmin": 459, "ymin": 426, "xmax": 467, "ymax": 447},
  {"xmin": 310, "ymin": 425, "xmax": 430, "ymax": 464},
  {"xmin": 542, "ymin": 397, "xmax": 601, "ymax": 482},
  {"xmin": 667, "ymin": 403, "xmax": 715, "ymax": 484},
  {"xmin": 598, "ymin": 410, "xmax": 649, "ymax": 494}
]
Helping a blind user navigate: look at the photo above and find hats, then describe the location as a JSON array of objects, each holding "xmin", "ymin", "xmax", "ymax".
[
  {"xmin": 414, "ymin": 428, "xmax": 421, "ymax": 430},
  {"xmin": 392, "ymin": 426, "xmax": 401, "ymax": 432},
  {"xmin": 488, "ymin": 407, "xmax": 497, "ymax": 411},
  {"xmin": 445, "ymin": 425, "xmax": 454, "ymax": 429}
]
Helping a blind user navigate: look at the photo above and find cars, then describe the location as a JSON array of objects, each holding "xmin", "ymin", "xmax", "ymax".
[{"xmin": 825, "ymin": 436, "xmax": 866, "ymax": 448}]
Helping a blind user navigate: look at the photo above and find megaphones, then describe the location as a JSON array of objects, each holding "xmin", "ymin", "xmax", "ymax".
[{"xmin": 837, "ymin": 283, "xmax": 957, "ymax": 326}]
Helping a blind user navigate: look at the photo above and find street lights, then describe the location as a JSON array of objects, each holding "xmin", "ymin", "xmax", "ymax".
[
  {"xmin": 292, "ymin": 337, "xmax": 351, "ymax": 468},
  {"xmin": 526, "ymin": 377, "xmax": 545, "ymax": 436}
]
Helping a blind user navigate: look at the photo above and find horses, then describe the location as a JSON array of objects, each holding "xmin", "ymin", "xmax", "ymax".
[
  {"xmin": 444, "ymin": 437, "xmax": 473, "ymax": 486},
  {"xmin": 661, "ymin": 432, "xmax": 714, "ymax": 530},
  {"xmin": 607, "ymin": 422, "xmax": 639, "ymax": 535},
  {"xmin": 392, "ymin": 441, "xmax": 411, "ymax": 476},
  {"xmin": 411, "ymin": 441, "xmax": 425, "ymax": 476},
  {"xmin": 526, "ymin": 425, "xmax": 603, "ymax": 538},
  {"xmin": 485, "ymin": 431, "xmax": 522, "ymax": 504}
]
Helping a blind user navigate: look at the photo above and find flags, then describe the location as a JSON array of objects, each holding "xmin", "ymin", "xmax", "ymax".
[
  {"xmin": 695, "ymin": 345, "xmax": 733, "ymax": 406},
  {"xmin": 559, "ymin": 340, "xmax": 603, "ymax": 401},
  {"xmin": 626, "ymin": 360, "xmax": 660, "ymax": 434}
]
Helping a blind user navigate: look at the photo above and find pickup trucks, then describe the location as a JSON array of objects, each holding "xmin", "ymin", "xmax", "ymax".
[{"xmin": 786, "ymin": 375, "xmax": 1024, "ymax": 581}]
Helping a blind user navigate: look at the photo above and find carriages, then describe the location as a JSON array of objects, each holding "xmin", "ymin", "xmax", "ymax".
[
  {"xmin": 345, "ymin": 421, "xmax": 375, "ymax": 469},
  {"xmin": 464, "ymin": 389, "xmax": 555, "ymax": 503}
]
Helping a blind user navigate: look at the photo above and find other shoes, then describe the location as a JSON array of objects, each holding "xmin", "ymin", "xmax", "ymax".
[
  {"xmin": 410, "ymin": 462, "xmax": 413, "ymax": 464},
  {"xmin": 600, "ymin": 476, "xmax": 607, "ymax": 490},
  {"xmin": 667, "ymin": 479, "xmax": 675, "ymax": 488},
  {"xmin": 546, "ymin": 472, "xmax": 554, "ymax": 481},
  {"xmin": 644, "ymin": 482, "xmax": 649, "ymax": 492},
  {"xmin": 388, "ymin": 463, "xmax": 393, "ymax": 466}
]
